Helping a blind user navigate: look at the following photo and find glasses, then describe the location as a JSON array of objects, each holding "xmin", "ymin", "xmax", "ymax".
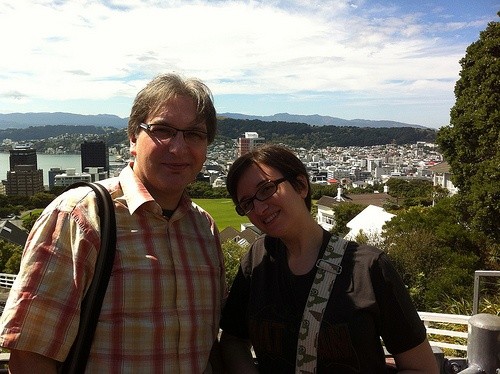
[
  {"xmin": 232, "ymin": 175, "xmax": 293, "ymax": 217},
  {"xmin": 136, "ymin": 120, "xmax": 209, "ymax": 146}
]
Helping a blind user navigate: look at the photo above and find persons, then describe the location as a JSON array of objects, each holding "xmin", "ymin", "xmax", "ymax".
[
  {"xmin": 213, "ymin": 145, "xmax": 439, "ymax": 374},
  {"xmin": 0, "ymin": 72, "xmax": 227, "ymax": 374}
]
